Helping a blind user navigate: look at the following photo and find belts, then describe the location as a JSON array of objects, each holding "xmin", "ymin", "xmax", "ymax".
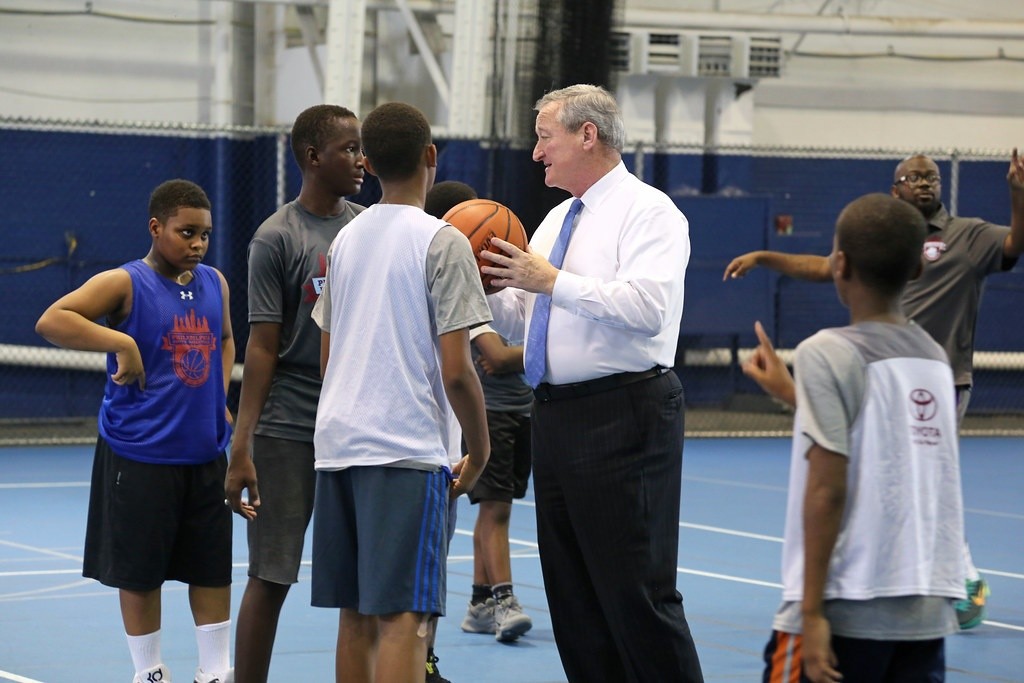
[{"xmin": 531, "ymin": 364, "xmax": 672, "ymax": 403}]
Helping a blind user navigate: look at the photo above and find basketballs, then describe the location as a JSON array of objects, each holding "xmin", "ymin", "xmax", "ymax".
[{"xmin": 438, "ymin": 197, "xmax": 530, "ymax": 296}]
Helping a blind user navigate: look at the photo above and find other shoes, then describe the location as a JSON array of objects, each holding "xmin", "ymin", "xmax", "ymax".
[
  {"xmin": 427, "ymin": 647, "xmax": 451, "ymax": 683},
  {"xmin": 193, "ymin": 667, "xmax": 220, "ymax": 683},
  {"xmin": 133, "ymin": 664, "xmax": 171, "ymax": 683},
  {"xmin": 952, "ymin": 578, "xmax": 988, "ymax": 629}
]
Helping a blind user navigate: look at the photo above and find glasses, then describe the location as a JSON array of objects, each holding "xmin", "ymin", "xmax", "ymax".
[{"xmin": 894, "ymin": 174, "xmax": 942, "ymax": 186}]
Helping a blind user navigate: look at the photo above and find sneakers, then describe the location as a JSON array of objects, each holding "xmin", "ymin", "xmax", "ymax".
[
  {"xmin": 492, "ymin": 595, "xmax": 533, "ymax": 642},
  {"xmin": 461, "ymin": 598, "xmax": 497, "ymax": 633}
]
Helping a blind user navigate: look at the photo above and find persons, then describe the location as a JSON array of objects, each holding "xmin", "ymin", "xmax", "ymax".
[
  {"xmin": 34, "ymin": 177, "xmax": 238, "ymax": 683},
  {"xmin": 725, "ymin": 150, "xmax": 1024, "ymax": 426},
  {"xmin": 214, "ymin": 69, "xmax": 709, "ymax": 683},
  {"xmin": 759, "ymin": 194, "xmax": 988, "ymax": 683}
]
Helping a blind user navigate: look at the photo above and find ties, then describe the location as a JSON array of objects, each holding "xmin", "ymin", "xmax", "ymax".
[{"xmin": 524, "ymin": 199, "xmax": 583, "ymax": 390}]
